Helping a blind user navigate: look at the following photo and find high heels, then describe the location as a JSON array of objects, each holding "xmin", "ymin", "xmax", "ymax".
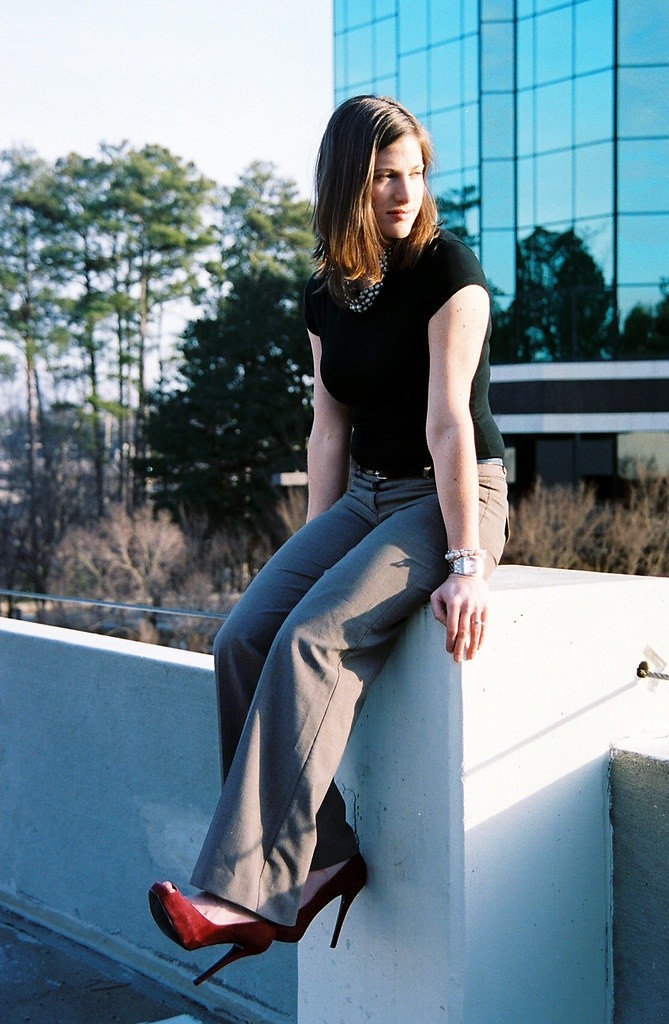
[
  {"xmin": 149, "ymin": 881, "xmax": 275, "ymax": 986},
  {"xmin": 273, "ymin": 853, "xmax": 368, "ymax": 948}
]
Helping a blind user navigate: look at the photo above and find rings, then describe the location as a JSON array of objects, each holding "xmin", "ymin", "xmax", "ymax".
[{"xmin": 472, "ymin": 621, "xmax": 480, "ymax": 624}]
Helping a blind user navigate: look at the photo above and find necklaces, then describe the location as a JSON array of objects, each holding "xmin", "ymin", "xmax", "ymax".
[{"xmin": 343, "ymin": 247, "xmax": 391, "ymax": 314}]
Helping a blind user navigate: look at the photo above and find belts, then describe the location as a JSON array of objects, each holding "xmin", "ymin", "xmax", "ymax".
[{"xmin": 358, "ymin": 466, "xmax": 435, "ymax": 482}]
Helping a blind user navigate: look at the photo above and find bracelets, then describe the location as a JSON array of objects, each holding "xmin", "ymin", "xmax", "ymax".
[{"xmin": 445, "ymin": 549, "xmax": 485, "ymax": 561}]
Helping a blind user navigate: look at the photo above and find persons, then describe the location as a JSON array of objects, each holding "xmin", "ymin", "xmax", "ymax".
[{"xmin": 150, "ymin": 94, "xmax": 508, "ymax": 984}]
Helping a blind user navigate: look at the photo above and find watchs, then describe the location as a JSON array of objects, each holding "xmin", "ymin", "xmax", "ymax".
[{"xmin": 449, "ymin": 556, "xmax": 483, "ymax": 576}]
